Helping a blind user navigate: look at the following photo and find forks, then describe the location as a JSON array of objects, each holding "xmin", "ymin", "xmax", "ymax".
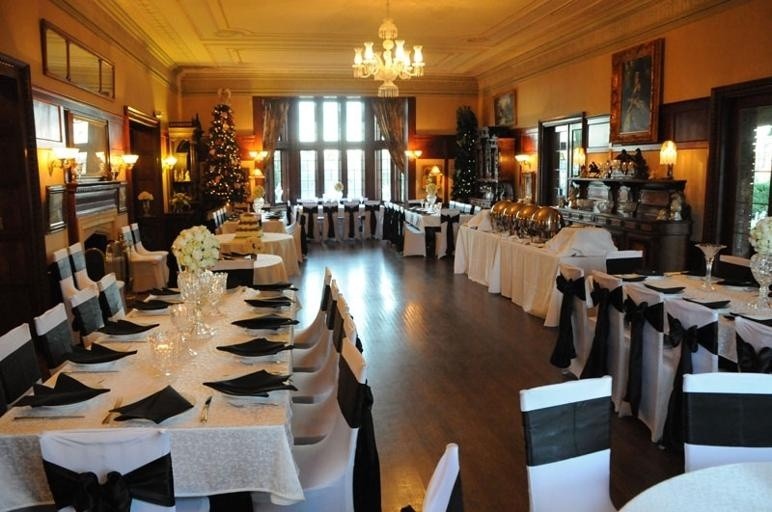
[{"xmin": 98, "ymin": 395, "xmax": 123, "ymax": 428}]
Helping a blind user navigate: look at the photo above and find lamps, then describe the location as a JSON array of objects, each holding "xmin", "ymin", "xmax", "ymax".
[
  {"xmin": 253, "ymin": 168, "xmax": 262, "ymax": 177},
  {"xmin": 67, "ymin": 147, "xmax": 79, "ymax": 168},
  {"xmin": 660, "ymin": 140, "xmax": 677, "ymax": 180},
  {"xmin": 573, "ymin": 147, "xmax": 586, "ymax": 172},
  {"xmin": 248, "ymin": 150, "xmax": 268, "ymax": 162},
  {"xmin": 118, "ymin": 154, "xmax": 139, "ymax": 172},
  {"xmin": 351, "ymin": 0, "xmax": 426, "ymax": 97},
  {"xmin": 95, "ymin": 152, "xmax": 105, "ymax": 164},
  {"xmin": 162, "ymin": 156, "xmax": 176, "ymax": 172},
  {"xmin": 405, "ymin": 148, "xmax": 422, "ymax": 159},
  {"xmin": 48, "ymin": 143, "xmax": 66, "ymax": 175},
  {"xmin": 431, "ymin": 161, "xmax": 440, "ymax": 173},
  {"xmin": 515, "ymin": 154, "xmax": 531, "ymax": 171}
]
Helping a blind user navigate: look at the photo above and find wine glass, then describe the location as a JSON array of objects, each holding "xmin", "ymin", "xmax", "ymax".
[
  {"xmin": 150, "ymin": 266, "xmax": 228, "ymax": 375},
  {"xmin": 420, "ymin": 198, "xmax": 442, "ymax": 216},
  {"xmin": 748, "ymin": 254, "xmax": 772, "ymax": 312},
  {"xmin": 696, "ymin": 243, "xmax": 725, "ymax": 291},
  {"xmin": 252, "ymin": 198, "xmax": 264, "ymax": 216}
]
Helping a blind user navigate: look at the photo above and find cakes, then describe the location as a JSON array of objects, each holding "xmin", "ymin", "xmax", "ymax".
[{"xmin": 234, "ymin": 212, "xmax": 263, "ymax": 238}]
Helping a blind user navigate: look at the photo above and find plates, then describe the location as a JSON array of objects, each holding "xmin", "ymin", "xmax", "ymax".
[
  {"xmin": 143, "ymin": 394, "xmax": 199, "ymax": 427},
  {"xmin": 38, "ymin": 291, "xmax": 180, "ymax": 413},
  {"xmin": 622, "ymin": 272, "xmax": 772, "ymax": 322},
  {"xmin": 222, "ymin": 289, "xmax": 293, "ymax": 406}
]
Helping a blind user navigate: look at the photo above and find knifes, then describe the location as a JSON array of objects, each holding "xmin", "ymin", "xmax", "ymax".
[{"xmin": 199, "ymin": 391, "xmax": 213, "ymax": 426}]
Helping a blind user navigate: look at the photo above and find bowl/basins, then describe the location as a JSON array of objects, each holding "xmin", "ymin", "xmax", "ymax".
[{"xmin": 576, "ymin": 199, "xmax": 638, "ymax": 216}]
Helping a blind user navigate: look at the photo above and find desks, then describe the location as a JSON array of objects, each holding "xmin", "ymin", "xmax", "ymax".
[{"xmin": 453, "ymin": 201, "xmax": 620, "ymax": 328}]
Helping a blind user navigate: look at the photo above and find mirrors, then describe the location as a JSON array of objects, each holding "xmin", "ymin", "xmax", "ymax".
[
  {"xmin": 100, "ymin": 53, "xmax": 116, "ymax": 103},
  {"xmin": 67, "ymin": 33, "xmax": 101, "ymax": 96},
  {"xmin": 41, "ymin": 18, "xmax": 69, "ymax": 83},
  {"xmin": 67, "ymin": 109, "xmax": 113, "ymax": 181}
]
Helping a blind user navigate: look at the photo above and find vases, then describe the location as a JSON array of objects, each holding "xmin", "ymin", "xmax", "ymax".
[{"xmin": 177, "ymin": 268, "xmax": 217, "ymax": 341}]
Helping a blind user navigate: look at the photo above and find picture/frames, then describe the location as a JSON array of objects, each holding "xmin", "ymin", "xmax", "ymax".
[
  {"xmin": 609, "ymin": 37, "xmax": 664, "ymax": 144},
  {"xmin": 491, "ymin": 88, "xmax": 518, "ymax": 126}
]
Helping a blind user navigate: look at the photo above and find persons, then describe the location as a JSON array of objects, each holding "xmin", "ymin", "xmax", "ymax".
[{"xmin": 622, "ymin": 71, "xmax": 649, "ymax": 132}]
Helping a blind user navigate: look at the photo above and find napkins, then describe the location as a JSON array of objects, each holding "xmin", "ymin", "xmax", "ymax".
[
  {"xmin": 11, "ymin": 371, "xmax": 113, "ymax": 408},
  {"xmin": 63, "ymin": 342, "xmax": 139, "ymax": 364},
  {"xmin": 229, "ymin": 313, "xmax": 302, "ymax": 330},
  {"xmin": 93, "ymin": 318, "xmax": 162, "ymax": 335},
  {"xmin": 203, "ymin": 369, "xmax": 299, "ymax": 397},
  {"xmin": 215, "ymin": 338, "xmax": 295, "ymax": 357},
  {"xmin": 137, "ymin": 288, "xmax": 181, "ymax": 299},
  {"xmin": 107, "ymin": 384, "xmax": 194, "ymax": 423},
  {"xmin": 124, "ymin": 297, "xmax": 174, "ymax": 313}
]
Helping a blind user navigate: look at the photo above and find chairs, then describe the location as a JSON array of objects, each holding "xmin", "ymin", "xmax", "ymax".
[
  {"xmin": 293, "ymin": 267, "xmax": 363, "ymax": 444},
  {"xmin": 68, "ymin": 285, "xmax": 105, "ymax": 346},
  {"xmin": 386, "ymin": 199, "xmax": 482, "ymax": 260},
  {"xmin": 67, "ymin": 242, "xmax": 123, "ymax": 295},
  {"xmin": 39, "ymin": 428, "xmax": 210, "ymax": 510},
  {"xmin": 121, "ymin": 223, "xmax": 170, "ymax": 293},
  {"xmin": 1, "ymin": 323, "xmax": 44, "ymax": 412},
  {"xmin": 520, "ymin": 374, "xmax": 618, "ymax": 511},
  {"xmin": 307, "ymin": 201, "xmax": 386, "ymax": 241},
  {"xmin": 97, "ymin": 270, "xmax": 126, "ymax": 324},
  {"xmin": 398, "ymin": 441, "xmax": 467, "ymax": 512},
  {"xmin": 51, "ymin": 247, "xmax": 81, "ymax": 337},
  {"xmin": 683, "ymin": 372, "xmax": 771, "ymax": 472},
  {"xmin": 549, "ymin": 250, "xmax": 771, "ymax": 451},
  {"xmin": 189, "ymin": 202, "xmax": 305, "ymax": 308},
  {"xmin": 34, "ymin": 303, "xmax": 75, "ymax": 376},
  {"xmin": 250, "ymin": 337, "xmax": 382, "ymax": 512}
]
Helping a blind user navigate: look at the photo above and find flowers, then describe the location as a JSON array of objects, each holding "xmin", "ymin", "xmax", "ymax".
[
  {"xmin": 426, "ymin": 183, "xmax": 437, "ymax": 196},
  {"xmin": 170, "ymin": 225, "xmax": 220, "ymax": 269},
  {"xmin": 252, "ymin": 185, "xmax": 265, "ymax": 198},
  {"xmin": 137, "ymin": 191, "xmax": 154, "ymax": 201},
  {"xmin": 335, "ymin": 183, "xmax": 344, "ymax": 192}
]
